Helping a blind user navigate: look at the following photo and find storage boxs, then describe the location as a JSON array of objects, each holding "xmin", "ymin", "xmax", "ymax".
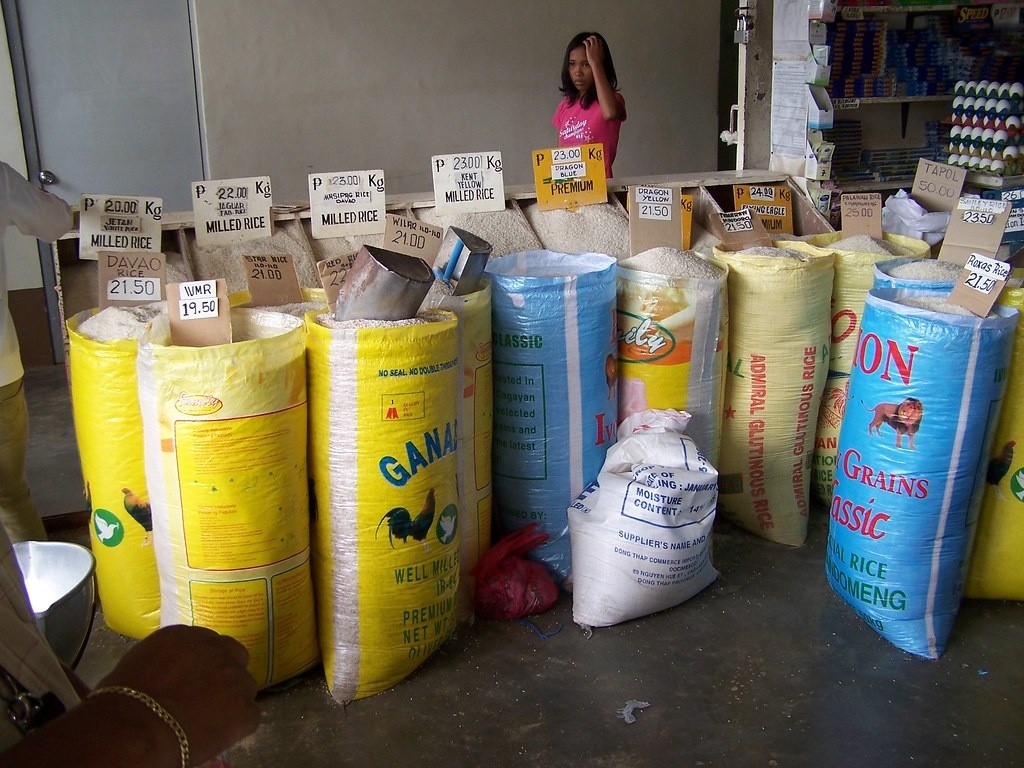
[
  {"xmin": 805, "ymin": 129, "xmax": 831, "ymax": 181},
  {"xmin": 805, "ymin": 84, "xmax": 833, "ymax": 129},
  {"xmin": 805, "ymin": 180, "xmax": 830, "ymax": 223},
  {"xmin": 806, "ymin": 44, "xmax": 830, "ymax": 86}
]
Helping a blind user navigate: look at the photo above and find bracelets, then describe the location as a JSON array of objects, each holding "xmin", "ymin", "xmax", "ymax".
[{"xmin": 87, "ymin": 685, "xmax": 189, "ymax": 768}]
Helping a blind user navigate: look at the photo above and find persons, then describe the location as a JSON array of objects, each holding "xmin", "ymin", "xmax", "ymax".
[
  {"xmin": 551, "ymin": 32, "xmax": 626, "ymax": 179},
  {"xmin": 0, "ymin": 625, "xmax": 263, "ymax": 768},
  {"xmin": 0, "ymin": 162, "xmax": 74, "ymax": 542}
]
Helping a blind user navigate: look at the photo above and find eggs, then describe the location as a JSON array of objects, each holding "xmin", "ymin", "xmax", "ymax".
[{"xmin": 947, "ymin": 81, "xmax": 1024, "ymax": 171}]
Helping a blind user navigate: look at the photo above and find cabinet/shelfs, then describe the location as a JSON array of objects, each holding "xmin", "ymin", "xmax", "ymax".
[{"xmin": 830, "ymin": 3, "xmax": 1024, "ymax": 193}]
